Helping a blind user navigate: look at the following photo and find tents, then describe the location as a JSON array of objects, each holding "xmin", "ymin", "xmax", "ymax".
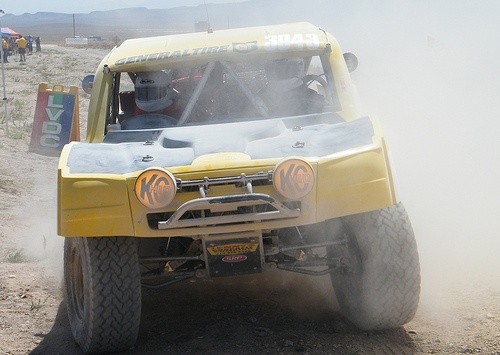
[{"xmin": 0, "ymin": 28, "xmax": 19, "ymax": 35}]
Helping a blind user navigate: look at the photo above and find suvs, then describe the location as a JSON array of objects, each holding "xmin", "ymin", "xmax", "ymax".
[{"xmin": 57, "ymin": 21, "xmax": 421, "ymax": 354}]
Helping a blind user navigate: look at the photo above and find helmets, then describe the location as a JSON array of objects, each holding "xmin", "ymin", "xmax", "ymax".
[
  {"xmin": 272, "ymin": 56, "xmax": 304, "ymax": 92},
  {"xmin": 135, "ymin": 71, "xmax": 172, "ymax": 112}
]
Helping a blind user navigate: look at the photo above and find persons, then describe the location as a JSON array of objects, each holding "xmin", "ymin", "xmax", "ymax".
[
  {"xmin": 241, "ymin": 53, "xmax": 327, "ymax": 119},
  {"xmin": 36, "ymin": 36, "xmax": 41, "ymax": 51},
  {"xmin": 2, "ymin": 36, "xmax": 10, "ymax": 63},
  {"xmin": 134, "ymin": 69, "xmax": 197, "ymax": 124},
  {"xmin": 1, "ymin": 35, "xmax": 33, "ymax": 55},
  {"xmin": 16, "ymin": 35, "xmax": 26, "ymax": 61}
]
